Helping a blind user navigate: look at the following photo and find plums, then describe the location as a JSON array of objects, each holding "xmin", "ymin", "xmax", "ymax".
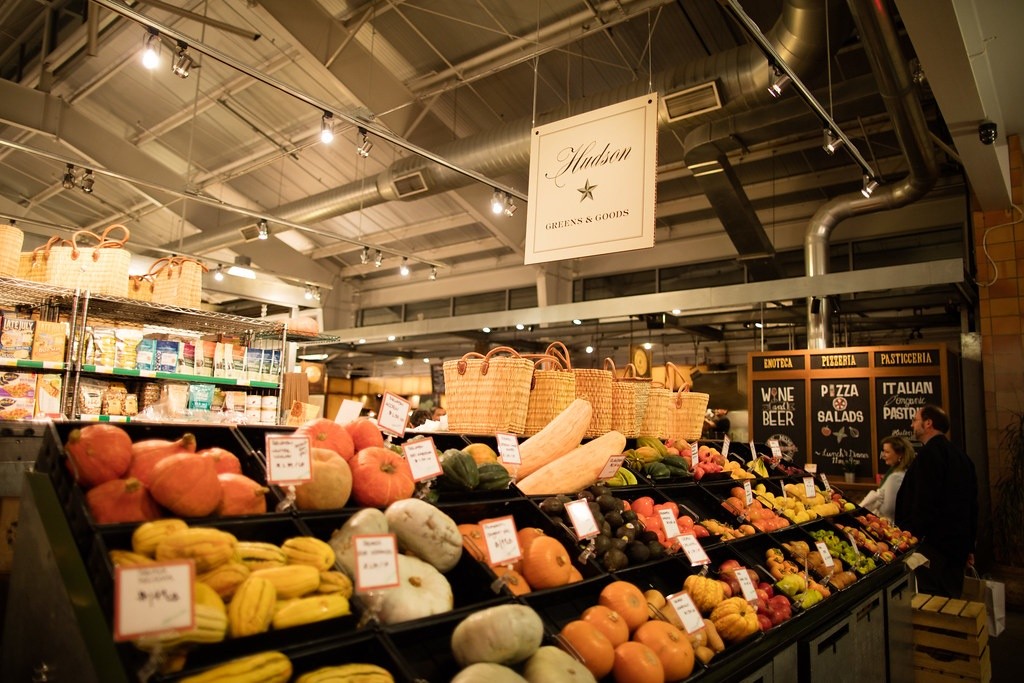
[{"xmin": 664, "ymin": 437, "xmax": 726, "ymax": 480}]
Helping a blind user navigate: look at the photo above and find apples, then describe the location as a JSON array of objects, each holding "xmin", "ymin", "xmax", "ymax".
[{"xmin": 836, "ymin": 515, "xmax": 917, "ymax": 562}]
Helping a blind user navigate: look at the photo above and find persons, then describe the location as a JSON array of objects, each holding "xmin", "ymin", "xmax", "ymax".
[
  {"xmin": 871, "ymin": 435, "xmax": 916, "ymax": 524},
  {"xmin": 410, "ymin": 406, "xmax": 446, "ymax": 428},
  {"xmin": 894, "ymin": 403, "xmax": 977, "ymax": 600}
]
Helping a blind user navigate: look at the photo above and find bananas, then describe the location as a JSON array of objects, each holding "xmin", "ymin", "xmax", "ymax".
[{"xmin": 747, "ymin": 457, "xmax": 769, "ymax": 478}]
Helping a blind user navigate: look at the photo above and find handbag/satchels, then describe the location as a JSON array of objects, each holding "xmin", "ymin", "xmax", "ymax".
[
  {"xmin": 517, "ymin": 350, "xmax": 577, "ymax": 437},
  {"xmin": 45, "ymin": 224, "xmax": 133, "ymax": 295},
  {"xmin": 600, "ymin": 357, "xmax": 652, "ymax": 440},
  {"xmin": 662, "ymin": 360, "xmax": 710, "ymax": 446},
  {"xmin": 438, "ymin": 347, "xmax": 532, "ymax": 436},
  {"xmin": 32, "ymin": 236, "xmax": 75, "ymax": 288},
  {"xmin": 646, "ymin": 380, "xmax": 678, "ymax": 444},
  {"xmin": 147, "ymin": 258, "xmax": 211, "ymax": 310},
  {"xmin": 546, "ymin": 340, "xmax": 616, "ymax": 442},
  {"xmin": 126, "ymin": 272, "xmax": 156, "ymax": 302}
]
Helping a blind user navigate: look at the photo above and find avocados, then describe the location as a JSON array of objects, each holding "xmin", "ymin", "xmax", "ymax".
[{"xmin": 541, "ymin": 487, "xmax": 665, "ymax": 573}]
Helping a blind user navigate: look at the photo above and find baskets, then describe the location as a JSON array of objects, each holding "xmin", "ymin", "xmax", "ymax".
[
  {"xmin": 0, "ymin": 224, "xmax": 26, "ymax": 279},
  {"xmin": 18, "ymin": 250, "xmax": 34, "ymax": 279}
]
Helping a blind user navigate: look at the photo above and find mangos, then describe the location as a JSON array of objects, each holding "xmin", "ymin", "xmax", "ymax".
[
  {"xmin": 620, "ymin": 496, "xmax": 709, "ymax": 553},
  {"xmin": 720, "ymin": 483, "xmax": 855, "ymax": 533},
  {"xmin": 715, "ymin": 560, "xmax": 790, "ymax": 630},
  {"xmin": 710, "ymin": 446, "xmax": 756, "ymax": 479}
]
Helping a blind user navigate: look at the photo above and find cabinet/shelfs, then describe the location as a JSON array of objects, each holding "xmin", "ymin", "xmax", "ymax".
[{"xmin": 0, "ymin": 277, "xmax": 919, "ymax": 683}]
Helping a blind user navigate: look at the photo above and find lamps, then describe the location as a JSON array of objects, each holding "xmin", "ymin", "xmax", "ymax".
[
  {"xmin": 215, "ymin": 256, "xmax": 321, "ymax": 300},
  {"xmin": 61, "ymin": 163, "xmax": 75, "ymax": 189},
  {"xmin": 142, "ymin": 27, "xmax": 161, "ymax": 68},
  {"xmin": 490, "ymin": 187, "xmax": 503, "ymax": 213},
  {"xmin": 373, "ymin": 249, "xmax": 383, "ymax": 268},
  {"xmin": 504, "ymin": 192, "xmax": 517, "ymax": 216},
  {"xmin": 172, "ymin": 40, "xmax": 193, "ymax": 78},
  {"xmin": 400, "ymin": 256, "xmax": 409, "ymax": 275},
  {"xmin": 360, "ymin": 246, "xmax": 370, "ymax": 264},
  {"xmin": 321, "ymin": 110, "xmax": 334, "ymax": 143},
  {"xmin": 357, "ymin": 126, "xmax": 373, "ymax": 157},
  {"xmin": 767, "ymin": 74, "xmax": 880, "ymax": 198},
  {"xmin": 81, "ymin": 168, "xmax": 94, "ymax": 194},
  {"xmin": 259, "ymin": 219, "xmax": 268, "ymax": 240},
  {"xmin": 429, "ymin": 265, "xmax": 437, "ymax": 280}
]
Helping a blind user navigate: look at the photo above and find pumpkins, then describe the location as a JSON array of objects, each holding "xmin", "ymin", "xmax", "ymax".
[
  {"xmin": 560, "ymin": 574, "xmax": 758, "ymax": 682},
  {"xmin": 498, "ymin": 397, "xmax": 626, "ymax": 495},
  {"xmin": 62, "ymin": 414, "xmax": 597, "ymax": 683},
  {"xmin": 621, "ymin": 435, "xmax": 695, "ymax": 480}
]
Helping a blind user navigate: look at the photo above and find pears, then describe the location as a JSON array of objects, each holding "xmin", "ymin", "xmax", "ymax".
[
  {"xmin": 775, "ymin": 572, "xmax": 823, "ymax": 609},
  {"xmin": 784, "ymin": 529, "xmax": 876, "ymax": 588}
]
